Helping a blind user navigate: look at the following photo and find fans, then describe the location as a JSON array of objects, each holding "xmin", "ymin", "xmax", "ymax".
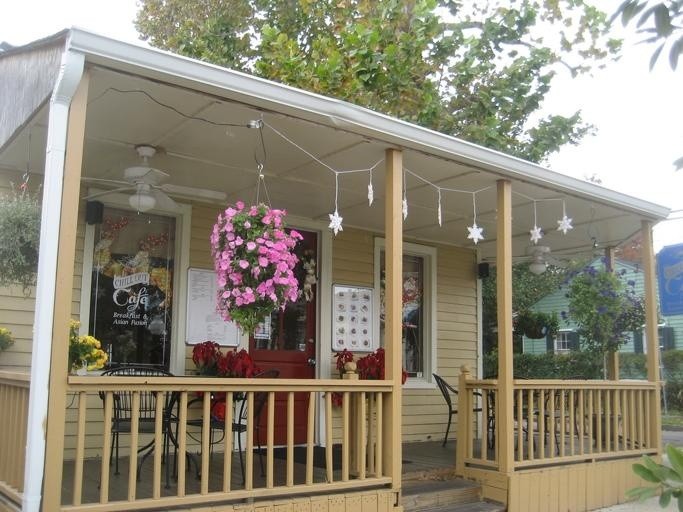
[
  {"xmin": 484, "ymin": 231, "xmax": 569, "ymax": 276},
  {"xmin": 80, "ymin": 144, "xmax": 227, "ymax": 223}
]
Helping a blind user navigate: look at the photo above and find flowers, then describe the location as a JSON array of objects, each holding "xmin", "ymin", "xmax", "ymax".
[
  {"xmin": 331, "ymin": 347, "xmax": 407, "ymax": 408},
  {"xmin": 67, "ymin": 318, "xmax": 108, "ymax": 374},
  {"xmin": 558, "ymin": 257, "xmax": 646, "ymax": 355},
  {"xmin": 0, "ymin": 327, "xmax": 14, "ymax": 351},
  {"xmin": 210, "ymin": 201, "xmax": 304, "ymax": 337},
  {"xmin": 192, "ymin": 340, "xmax": 264, "ymax": 422}
]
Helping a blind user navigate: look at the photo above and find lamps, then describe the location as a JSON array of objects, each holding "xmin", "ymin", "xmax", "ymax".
[
  {"xmin": 127, "ymin": 190, "xmax": 155, "ymax": 215},
  {"xmin": 527, "ymin": 261, "xmax": 545, "ymax": 275}
]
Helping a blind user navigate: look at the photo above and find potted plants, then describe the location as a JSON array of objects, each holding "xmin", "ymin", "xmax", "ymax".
[{"xmin": 514, "ymin": 304, "xmax": 559, "ymax": 340}]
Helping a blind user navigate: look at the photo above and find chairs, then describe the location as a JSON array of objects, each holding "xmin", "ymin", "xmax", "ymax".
[
  {"xmin": 430, "ymin": 372, "xmax": 587, "ymax": 457},
  {"xmin": 96, "ymin": 366, "xmax": 278, "ymax": 489}
]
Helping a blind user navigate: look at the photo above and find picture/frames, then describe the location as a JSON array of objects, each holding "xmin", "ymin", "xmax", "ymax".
[
  {"xmin": 330, "ymin": 283, "xmax": 376, "ymax": 352},
  {"xmin": 185, "ymin": 266, "xmax": 241, "ymax": 347}
]
